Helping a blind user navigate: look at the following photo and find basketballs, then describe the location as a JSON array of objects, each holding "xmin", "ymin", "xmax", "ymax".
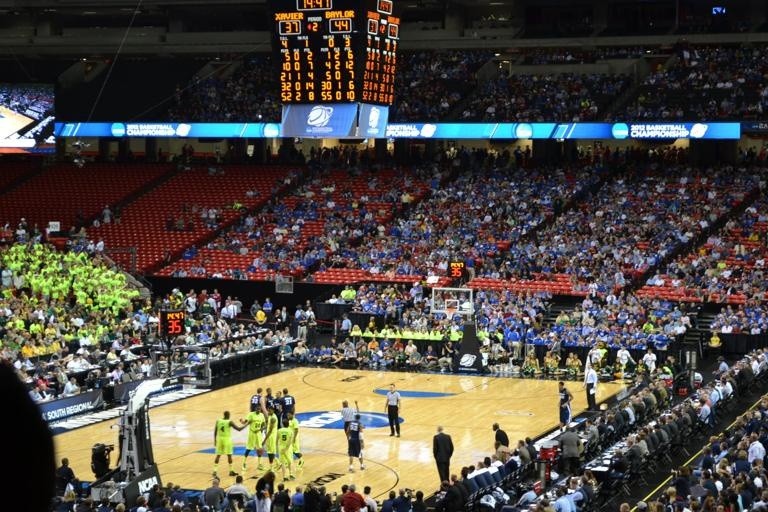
[{"xmin": 257, "ymin": 448, "xmax": 265, "ymax": 457}]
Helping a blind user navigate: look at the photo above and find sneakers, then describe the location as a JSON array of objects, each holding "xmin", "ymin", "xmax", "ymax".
[
  {"xmin": 360, "ymin": 464, "xmax": 365, "ymax": 470},
  {"xmin": 349, "ymin": 465, "xmax": 353, "ymax": 471},
  {"xmin": 229, "ymin": 471, "xmax": 239, "ymax": 476},
  {"xmin": 256, "ymin": 457, "xmax": 281, "ymax": 473},
  {"xmin": 212, "ymin": 471, "xmax": 220, "ymax": 481},
  {"xmin": 289, "ymin": 471, "xmax": 296, "ymax": 480},
  {"xmin": 296, "ymin": 458, "xmax": 304, "ymax": 469},
  {"xmin": 242, "ymin": 464, "xmax": 247, "ymax": 471},
  {"xmin": 283, "ymin": 475, "xmax": 289, "ymax": 481},
  {"xmin": 390, "ymin": 433, "xmax": 395, "ymax": 436},
  {"xmin": 395, "ymin": 435, "xmax": 400, "ymax": 438}
]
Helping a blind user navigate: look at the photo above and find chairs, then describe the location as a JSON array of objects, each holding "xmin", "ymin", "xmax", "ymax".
[
  {"xmin": 633, "ymin": 220, "xmax": 767, "ymax": 303},
  {"xmin": 466, "ymin": 270, "xmax": 579, "ymax": 295}
]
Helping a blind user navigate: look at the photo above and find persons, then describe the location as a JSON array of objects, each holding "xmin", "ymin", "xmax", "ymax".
[{"xmin": 0, "ymin": 38, "xmax": 768, "ymax": 510}]
